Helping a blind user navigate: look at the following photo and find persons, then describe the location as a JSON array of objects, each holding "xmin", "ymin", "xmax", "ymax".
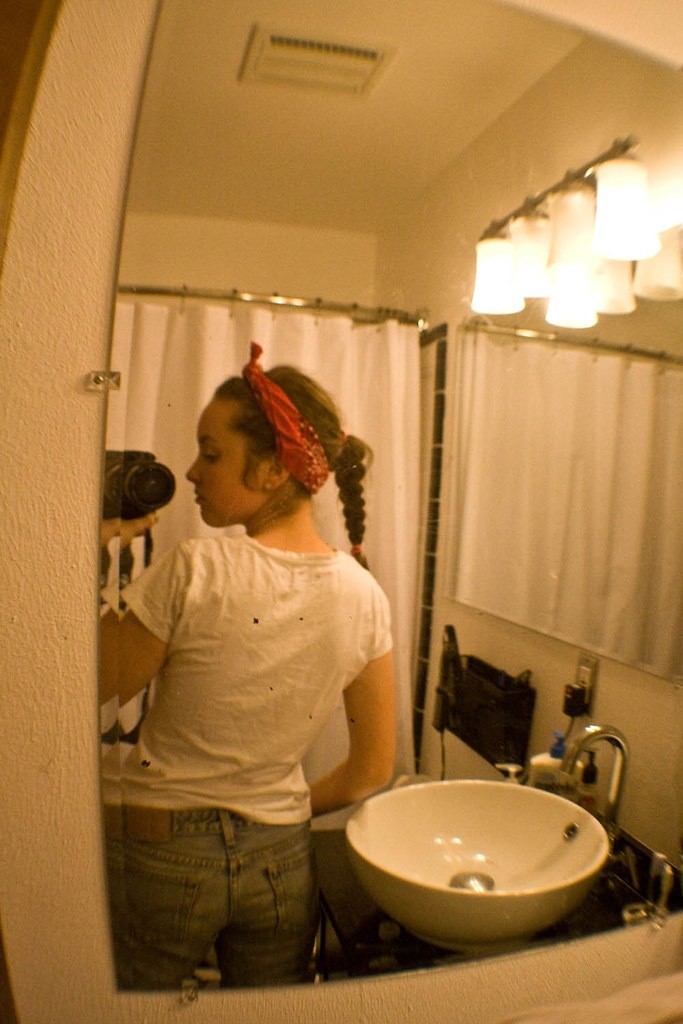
[{"xmin": 97, "ymin": 340, "xmax": 398, "ymax": 991}]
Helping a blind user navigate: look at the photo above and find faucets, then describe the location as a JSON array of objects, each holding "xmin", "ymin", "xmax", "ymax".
[{"xmin": 559, "ymin": 724, "xmax": 631, "ymax": 840}]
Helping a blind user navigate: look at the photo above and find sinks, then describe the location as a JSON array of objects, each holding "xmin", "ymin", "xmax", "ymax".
[{"xmin": 346, "ymin": 779, "xmax": 609, "ymax": 946}]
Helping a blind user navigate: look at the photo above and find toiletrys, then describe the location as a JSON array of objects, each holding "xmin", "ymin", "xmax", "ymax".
[
  {"xmin": 531, "ymin": 731, "xmax": 583, "ymax": 799},
  {"xmin": 580, "ymin": 747, "xmax": 600, "ymax": 818},
  {"xmin": 495, "ymin": 762, "xmax": 523, "ymax": 783}
]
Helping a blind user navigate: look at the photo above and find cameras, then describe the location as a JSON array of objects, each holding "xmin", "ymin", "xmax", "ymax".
[{"xmin": 102, "ymin": 450, "xmax": 175, "ymax": 523}]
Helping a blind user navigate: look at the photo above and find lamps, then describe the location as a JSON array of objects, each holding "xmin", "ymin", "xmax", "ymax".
[
  {"xmin": 545, "ymin": 231, "xmax": 683, "ymax": 331},
  {"xmin": 467, "ymin": 135, "xmax": 663, "ymax": 315}
]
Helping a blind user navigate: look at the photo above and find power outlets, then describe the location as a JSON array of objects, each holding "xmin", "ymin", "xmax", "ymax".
[{"xmin": 574, "ymin": 652, "xmax": 601, "ymax": 719}]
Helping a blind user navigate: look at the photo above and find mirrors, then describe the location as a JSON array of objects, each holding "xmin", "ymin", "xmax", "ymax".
[
  {"xmin": 435, "ymin": 222, "xmax": 683, "ymax": 687},
  {"xmin": 91, "ymin": 0, "xmax": 683, "ymax": 1000}
]
